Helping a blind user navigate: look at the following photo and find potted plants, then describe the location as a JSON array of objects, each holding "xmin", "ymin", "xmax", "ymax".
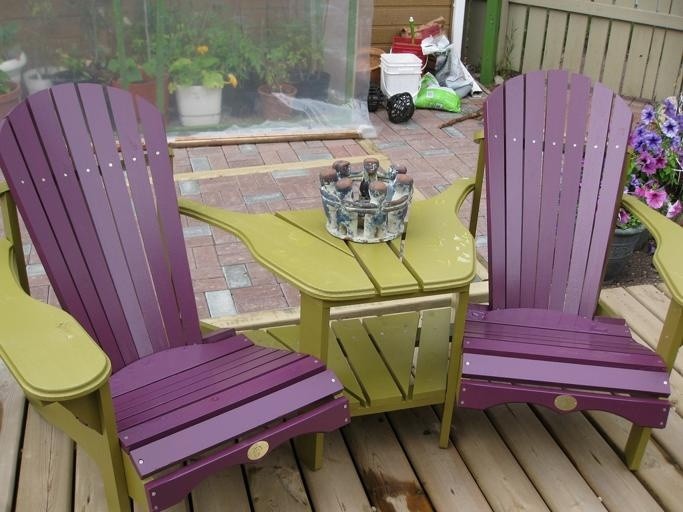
[{"xmin": 0, "ymin": 1, "xmax": 331, "ymax": 127}]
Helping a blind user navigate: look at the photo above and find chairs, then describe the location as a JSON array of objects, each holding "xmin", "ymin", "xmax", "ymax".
[
  {"xmin": 1, "ymin": 83, "xmax": 350, "ymax": 510},
  {"xmin": 456, "ymin": 72, "xmax": 682, "ymax": 473}
]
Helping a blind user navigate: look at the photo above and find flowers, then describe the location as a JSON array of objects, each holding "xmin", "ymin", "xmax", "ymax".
[{"xmin": 611, "ymin": 94, "xmax": 683, "ymax": 231}]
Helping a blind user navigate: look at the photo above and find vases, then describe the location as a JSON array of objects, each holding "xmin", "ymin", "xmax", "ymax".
[{"xmin": 602, "ymin": 224, "xmax": 649, "ymax": 287}]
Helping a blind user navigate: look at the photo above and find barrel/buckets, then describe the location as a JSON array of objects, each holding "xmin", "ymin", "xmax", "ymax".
[
  {"xmin": 389, "ymin": 36, "xmax": 429, "ymax": 74},
  {"xmin": 379, "ymin": 53, "xmax": 422, "ymax": 101}
]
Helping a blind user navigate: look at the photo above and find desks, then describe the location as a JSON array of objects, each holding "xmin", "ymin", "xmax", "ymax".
[{"xmin": 177, "ymin": 175, "xmax": 477, "ymax": 472}]
